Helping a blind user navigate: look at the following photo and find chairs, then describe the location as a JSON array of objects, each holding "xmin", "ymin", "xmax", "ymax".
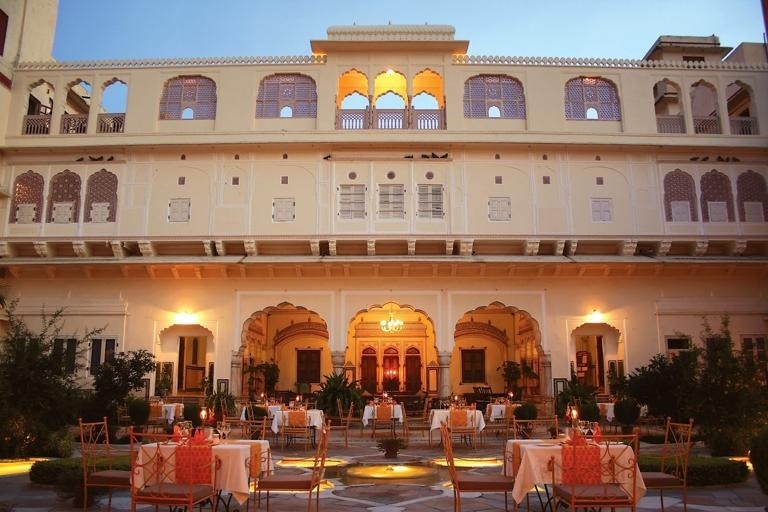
[
  {"xmin": 640, "ymin": 417, "xmax": 694, "ymax": 511},
  {"xmin": 218, "ymin": 415, "xmax": 267, "ymax": 511},
  {"xmin": 76, "ymin": 417, "xmax": 159, "ymax": 512},
  {"xmin": 439, "ymin": 421, "xmax": 515, "ymax": 511},
  {"xmin": 255, "ymin": 429, "xmax": 329, "ymax": 512},
  {"xmin": 128, "ymin": 425, "xmax": 219, "ymax": 512},
  {"xmin": 510, "ymin": 415, "xmax": 559, "ymax": 511},
  {"xmin": 114, "ymin": 387, "xmax": 673, "ymax": 451},
  {"xmin": 548, "ymin": 426, "xmax": 640, "ymax": 511}
]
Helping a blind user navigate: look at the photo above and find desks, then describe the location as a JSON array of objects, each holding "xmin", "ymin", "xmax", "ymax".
[
  {"xmin": 502, "ymin": 438, "xmax": 647, "ymax": 511},
  {"xmin": 129, "ymin": 437, "xmax": 275, "ymax": 512}
]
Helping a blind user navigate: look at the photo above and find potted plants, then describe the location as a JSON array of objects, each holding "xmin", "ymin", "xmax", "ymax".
[{"xmin": 311, "ymin": 365, "xmax": 366, "ymax": 426}]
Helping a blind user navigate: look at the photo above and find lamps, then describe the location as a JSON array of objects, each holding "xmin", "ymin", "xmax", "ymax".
[{"xmin": 380, "ymin": 301, "xmax": 404, "ymax": 335}]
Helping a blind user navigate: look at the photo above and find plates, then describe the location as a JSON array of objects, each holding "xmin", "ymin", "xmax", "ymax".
[
  {"xmin": 536, "ymin": 443, "xmax": 554, "ymax": 446},
  {"xmin": 600, "ymin": 441, "xmax": 623, "ymax": 445}
]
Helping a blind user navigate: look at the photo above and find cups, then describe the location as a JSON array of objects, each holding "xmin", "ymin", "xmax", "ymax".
[
  {"xmin": 282, "ymin": 404, "xmax": 288, "ymax": 410},
  {"xmin": 495, "ymin": 399, "xmax": 500, "ymax": 404},
  {"xmin": 373, "ymin": 396, "xmax": 392, "ymax": 404},
  {"xmin": 265, "ymin": 399, "xmax": 275, "ymax": 406}
]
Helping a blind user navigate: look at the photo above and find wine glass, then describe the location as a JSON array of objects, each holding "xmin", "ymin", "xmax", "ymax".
[
  {"xmin": 164, "ymin": 398, "xmax": 167, "ymax": 405},
  {"xmin": 576, "ymin": 419, "xmax": 597, "ymax": 445},
  {"xmin": 175, "ymin": 419, "xmax": 231, "ymax": 445},
  {"xmin": 288, "ymin": 400, "xmax": 294, "ymax": 411},
  {"xmin": 443, "ymin": 399, "xmax": 465, "ymax": 410}
]
